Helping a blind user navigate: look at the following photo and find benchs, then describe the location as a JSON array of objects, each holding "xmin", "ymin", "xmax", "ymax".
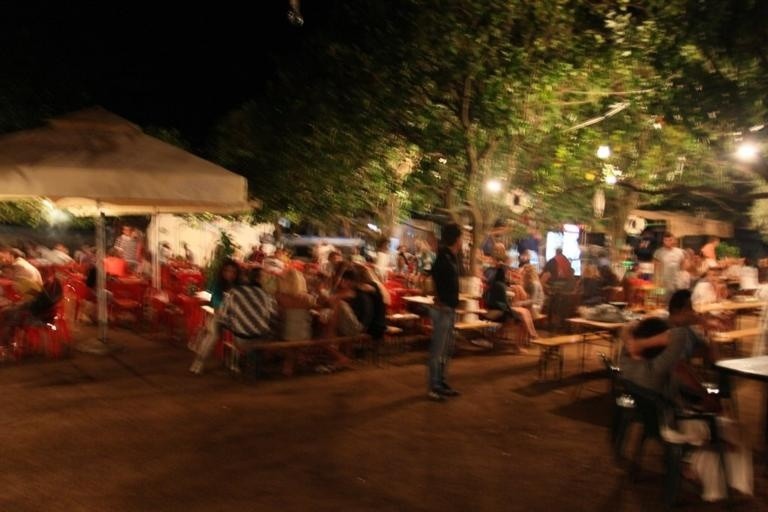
[
  {"xmin": 1, "ymin": 246, "xmax": 522, "ymax": 377},
  {"xmin": 523, "ymin": 247, "xmax": 768, "ymax": 506}
]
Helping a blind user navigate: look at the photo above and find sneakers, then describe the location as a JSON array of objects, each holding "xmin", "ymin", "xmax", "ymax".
[{"xmin": 428, "ymin": 384, "xmax": 460, "ymax": 402}]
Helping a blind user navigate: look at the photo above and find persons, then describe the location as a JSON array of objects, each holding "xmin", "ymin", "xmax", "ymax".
[
  {"xmin": 2, "ymin": 223, "xmax": 390, "ymax": 382},
  {"xmin": 399, "ymin": 222, "xmax": 767, "ymax": 511}
]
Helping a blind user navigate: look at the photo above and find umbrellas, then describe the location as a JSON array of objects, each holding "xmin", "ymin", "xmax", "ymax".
[{"xmin": 0, "ymin": 104, "xmax": 254, "ymax": 343}]
